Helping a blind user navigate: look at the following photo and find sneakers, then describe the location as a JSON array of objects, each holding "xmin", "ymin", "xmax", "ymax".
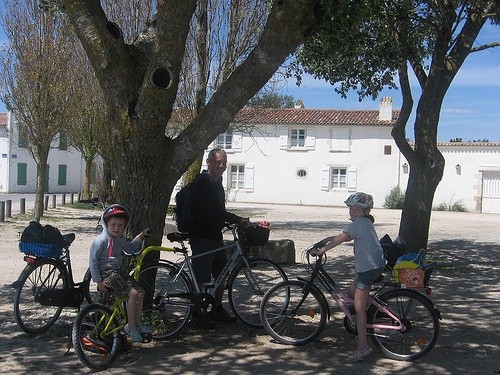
[
  {"xmin": 191, "ymin": 309, "xmax": 238, "ymax": 330},
  {"xmin": 124, "ymin": 324, "xmax": 153, "ymax": 342}
]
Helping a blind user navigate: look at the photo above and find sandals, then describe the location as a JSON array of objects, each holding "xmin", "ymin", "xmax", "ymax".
[{"xmin": 350, "ymin": 346, "xmax": 373, "ymax": 362}]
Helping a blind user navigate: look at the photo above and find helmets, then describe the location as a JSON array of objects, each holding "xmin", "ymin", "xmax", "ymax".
[{"xmin": 344, "ymin": 192, "xmax": 373, "ymax": 209}]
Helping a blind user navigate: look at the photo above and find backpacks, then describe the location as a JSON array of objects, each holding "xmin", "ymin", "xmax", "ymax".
[{"xmin": 175, "ymin": 173, "xmax": 202, "ymax": 232}]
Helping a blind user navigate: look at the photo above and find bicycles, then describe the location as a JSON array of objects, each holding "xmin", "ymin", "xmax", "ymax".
[
  {"xmin": 11, "ymin": 191, "xmax": 100, "ymax": 334},
  {"xmin": 64, "ymin": 227, "xmax": 152, "ymax": 371},
  {"xmin": 259, "ymin": 243, "xmax": 442, "ymax": 362},
  {"xmin": 129, "ymin": 220, "xmax": 290, "ymax": 340}
]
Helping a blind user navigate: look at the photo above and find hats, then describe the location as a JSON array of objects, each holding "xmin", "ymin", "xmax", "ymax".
[{"xmin": 103, "ymin": 208, "xmax": 129, "ymax": 219}]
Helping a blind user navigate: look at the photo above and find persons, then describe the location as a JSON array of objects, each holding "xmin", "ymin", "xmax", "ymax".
[
  {"xmin": 175, "ymin": 149, "xmax": 249, "ymax": 331},
  {"xmin": 309, "ymin": 192, "xmax": 386, "ymax": 362},
  {"xmin": 89, "ymin": 203, "xmax": 154, "ymax": 342}
]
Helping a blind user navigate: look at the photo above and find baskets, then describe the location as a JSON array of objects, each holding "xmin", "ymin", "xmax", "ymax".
[
  {"xmin": 19, "ymin": 242, "xmax": 62, "ymax": 258},
  {"xmin": 238, "ymin": 221, "xmax": 271, "ymax": 245},
  {"xmin": 395, "ymin": 268, "xmax": 433, "ymax": 289}
]
